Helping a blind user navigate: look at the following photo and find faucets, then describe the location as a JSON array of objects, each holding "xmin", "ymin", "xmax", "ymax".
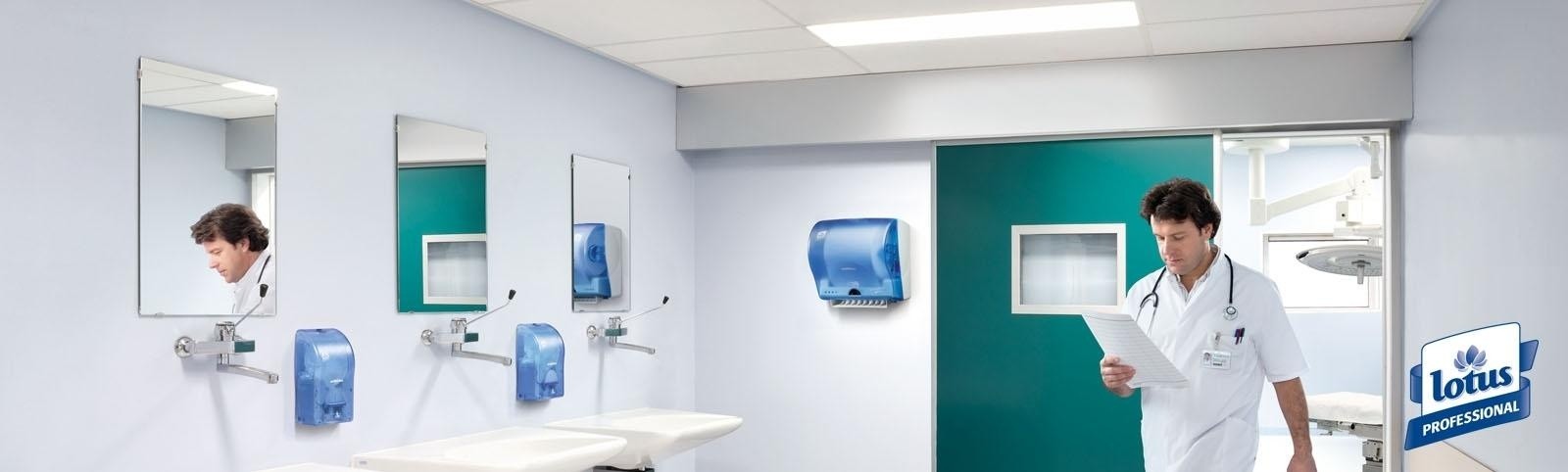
[
  {"xmin": 586, "ymin": 316, "xmax": 655, "ymax": 354},
  {"xmin": 174, "ymin": 322, "xmax": 279, "ymax": 385},
  {"xmin": 421, "ymin": 319, "xmax": 512, "ymax": 366}
]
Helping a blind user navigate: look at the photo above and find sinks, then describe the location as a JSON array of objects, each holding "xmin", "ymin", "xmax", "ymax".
[
  {"xmin": 544, "ymin": 410, "xmax": 743, "ymax": 470},
  {"xmin": 352, "ymin": 425, "xmax": 628, "ymax": 472}
]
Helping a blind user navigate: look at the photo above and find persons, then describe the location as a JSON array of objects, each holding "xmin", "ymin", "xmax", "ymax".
[
  {"xmin": 1096, "ymin": 176, "xmax": 1320, "ymax": 472},
  {"xmin": 188, "ymin": 202, "xmax": 275, "ymax": 318}
]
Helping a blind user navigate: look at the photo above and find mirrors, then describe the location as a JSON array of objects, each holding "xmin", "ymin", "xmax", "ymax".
[
  {"xmin": 396, "ymin": 114, "xmax": 488, "ymax": 314},
  {"xmin": 137, "ymin": 57, "xmax": 279, "ymax": 318},
  {"xmin": 570, "ymin": 153, "xmax": 631, "ymax": 312}
]
